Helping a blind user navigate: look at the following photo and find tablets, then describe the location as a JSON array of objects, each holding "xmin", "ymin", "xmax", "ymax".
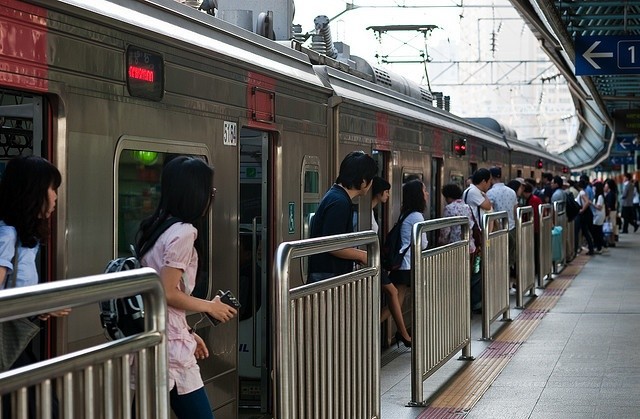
[{"xmin": 203, "ymin": 290, "xmax": 242, "ymax": 328}]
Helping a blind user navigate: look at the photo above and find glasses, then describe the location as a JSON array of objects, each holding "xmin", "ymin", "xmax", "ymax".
[{"xmin": 209, "ymin": 187, "xmax": 217, "ymax": 199}]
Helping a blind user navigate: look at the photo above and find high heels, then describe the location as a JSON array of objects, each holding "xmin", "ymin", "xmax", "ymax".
[{"xmin": 395, "ymin": 331, "xmax": 412, "ymax": 348}]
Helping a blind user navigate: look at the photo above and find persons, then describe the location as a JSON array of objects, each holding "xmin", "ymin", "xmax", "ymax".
[
  {"xmin": 0, "ymin": 154, "xmax": 72, "ymax": 419},
  {"xmin": 618, "ymin": 172, "xmax": 640, "ymax": 234},
  {"xmin": 123, "ymin": 154, "xmax": 237, "ymax": 419},
  {"xmin": 381, "ymin": 178, "xmax": 429, "ymax": 323},
  {"xmin": 485, "ymin": 167, "xmax": 519, "ymax": 267},
  {"xmin": 504, "ymin": 180, "xmax": 522, "ymax": 296},
  {"xmin": 352, "ymin": 175, "xmax": 413, "ymax": 350},
  {"xmin": 304, "ymin": 149, "xmax": 380, "ymax": 285},
  {"xmin": 515, "ymin": 172, "xmax": 619, "ymax": 256},
  {"xmin": 436, "ymin": 183, "xmax": 475, "ymax": 278},
  {"xmin": 463, "ymin": 168, "xmax": 494, "ymax": 247}
]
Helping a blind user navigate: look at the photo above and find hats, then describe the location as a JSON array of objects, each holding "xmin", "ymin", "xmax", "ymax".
[
  {"xmin": 490, "ymin": 168, "xmax": 501, "ymax": 178},
  {"xmin": 515, "ymin": 178, "xmax": 526, "ymax": 184}
]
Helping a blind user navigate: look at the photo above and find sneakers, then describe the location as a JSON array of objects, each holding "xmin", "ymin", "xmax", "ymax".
[
  {"xmin": 586, "ymin": 251, "xmax": 594, "ymax": 255},
  {"xmin": 634, "ymin": 225, "xmax": 639, "ymax": 232},
  {"xmin": 619, "ymin": 232, "xmax": 628, "ymax": 234},
  {"xmin": 594, "ymin": 249, "xmax": 602, "ymax": 254}
]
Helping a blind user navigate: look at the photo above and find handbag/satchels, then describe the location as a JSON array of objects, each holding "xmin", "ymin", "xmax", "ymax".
[
  {"xmin": 602, "ymin": 216, "xmax": 613, "ymax": 241},
  {"xmin": 582, "ymin": 206, "xmax": 593, "ymax": 224},
  {"xmin": 0, "ymin": 317, "xmax": 40, "ymax": 373},
  {"xmin": 551, "ymin": 226, "xmax": 562, "ymax": 260},
  {"xmin": 472, "ymin": 221, "xmax": 480, "ymax": 238}
]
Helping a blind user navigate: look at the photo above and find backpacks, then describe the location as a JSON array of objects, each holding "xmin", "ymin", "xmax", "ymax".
[
  {"xmin": 565, "ymin": 192, "xmax": 580, "ymax": 222},
  {"xmin": 380, "ymin": 212, "xmax": 411, "ymax": 271},
  {"xmin": 100, "ymin": 217, "xmax": 183, "ymax": 341}
]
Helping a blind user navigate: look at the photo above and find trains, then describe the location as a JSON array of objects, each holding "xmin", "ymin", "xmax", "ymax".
[{"xmin": 0, "ymin": 1, "xmax": 572, "ymax": 419}]
